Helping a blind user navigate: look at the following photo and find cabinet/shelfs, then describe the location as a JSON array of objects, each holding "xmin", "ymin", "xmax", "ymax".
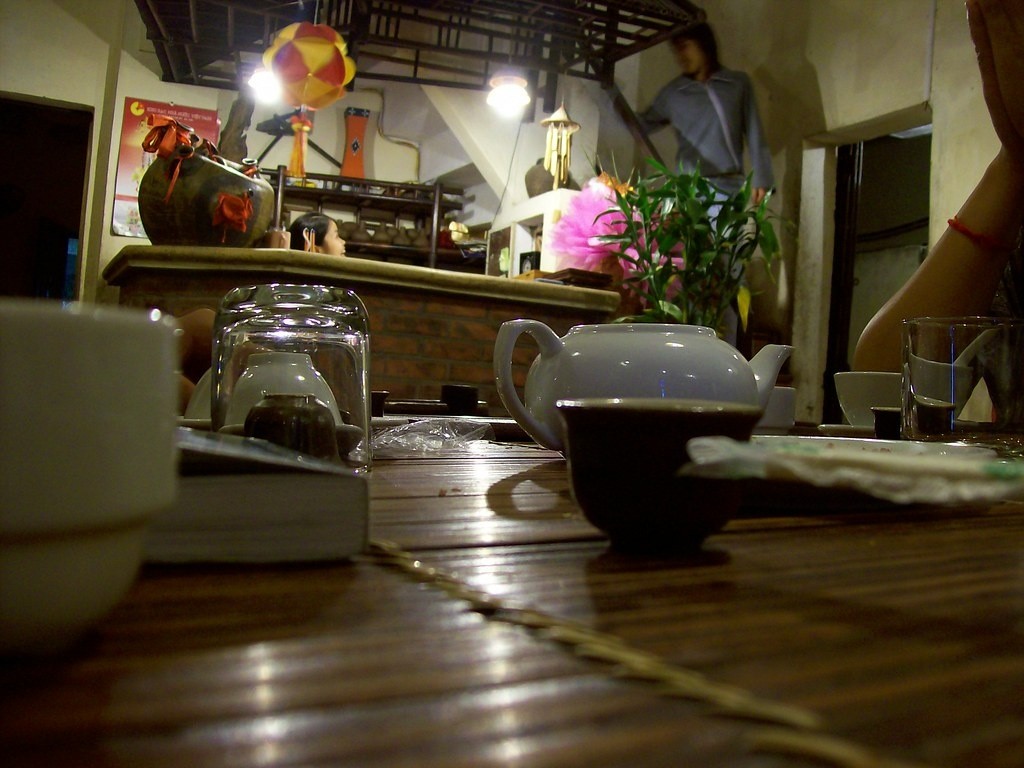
[{"xmin": 262, "ymin": 165, "xmax": 464, "ymax": 269}]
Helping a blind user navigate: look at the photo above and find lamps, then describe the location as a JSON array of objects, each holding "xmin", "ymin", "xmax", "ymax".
[{"xmin": 486, "ymin": 58, "xmax": 533, "ymax": 113}]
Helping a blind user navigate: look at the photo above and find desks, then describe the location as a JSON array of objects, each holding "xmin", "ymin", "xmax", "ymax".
[{"xmin": 0, "ymin": 439, "xmax": 1023, "ymax": 768}]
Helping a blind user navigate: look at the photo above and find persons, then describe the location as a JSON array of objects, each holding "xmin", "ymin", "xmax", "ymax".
[
  {"xmin": 288, "ymin": 212, "xmax": 346, "ymax": 256},
  {"xmin": 609, "ymin": 25, "xmax": 775, "ymax": 235},
  {"xmin": 848, "ymin": 0, "xmax": 1024, "ymax": 426}
]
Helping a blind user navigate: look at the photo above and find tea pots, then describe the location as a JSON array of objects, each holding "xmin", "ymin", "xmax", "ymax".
[{"xmin": 493, "ymin": 317, "xmax": 795, "ymax": 463}]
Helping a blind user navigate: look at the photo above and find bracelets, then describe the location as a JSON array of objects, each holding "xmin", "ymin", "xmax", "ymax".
[{"xmin": 946, "ymin": 216, "xmax": 1012, "ymax": 255}]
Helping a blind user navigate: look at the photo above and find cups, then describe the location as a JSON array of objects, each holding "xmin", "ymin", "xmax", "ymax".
[
  {"xmin": 901, "ymin": 316, "xmax": 1010, "ymax": 444},
  {"xmin": 0, "ymin": 300, "xmax": 184, "ymax": 640},
  {"xmin": 833, "ymin": 371, "xmax": 902, "ymax": 427},
  {"xmin": 209, "ymin": 282, "xmax": 374, "ymax": 474},
  {"xmin": 244, "ymin": 392, "xmax": 343, "ymax": 468},
  {"xmin": 870, "ymin": 406, "xmax": 903, "ymax": 440},
  {"xmin": 914, "ymin": 394, "xmax": 956, "ymax": 435},
  {"xmin": 555, "ymin": 396, "xmax": 769, "ymax": 569},
  {"xmin": 214, "ymin": 349, "xmax": 366, "ymax": 460},
  {"xmin": 750, "ymin": 385, "xmax": 796, "ymax": 437}
]
